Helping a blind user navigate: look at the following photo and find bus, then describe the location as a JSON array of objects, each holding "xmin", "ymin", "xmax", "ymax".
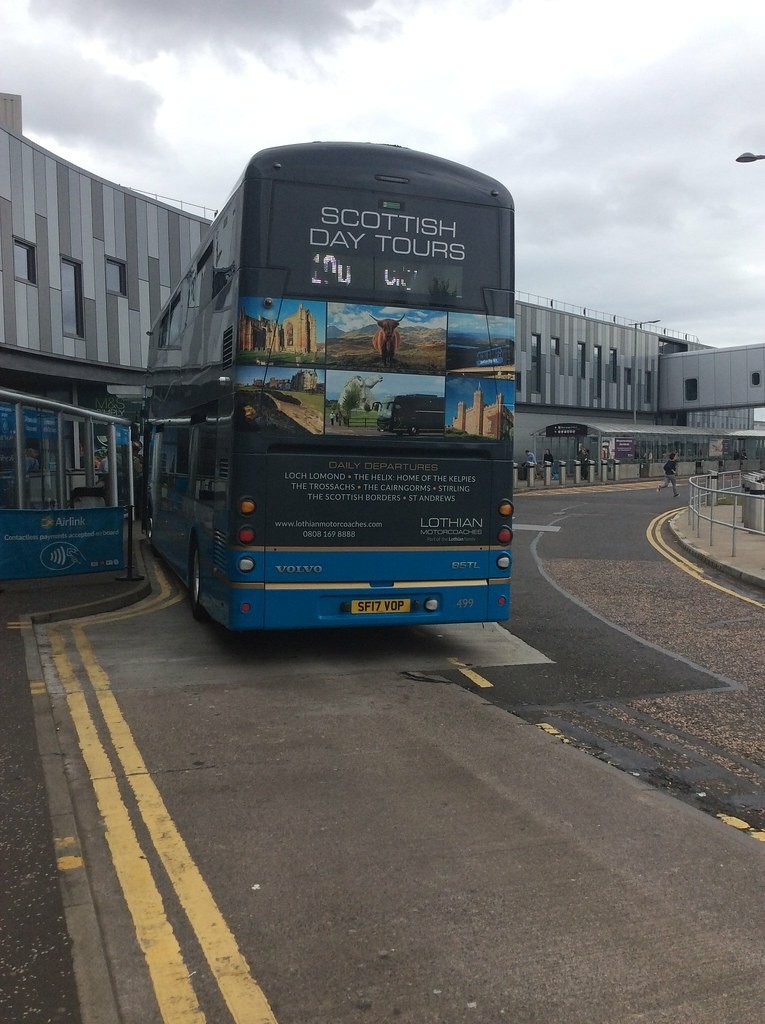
[
  {"xmin": 372, "ymin": 394, "xmax": 445, "ymax": 437},
  {"xmin": 475, "ymin": 345, "xmax": 513, "ymax": 367},
  {"xmin": 141, "ymin": 142, "xmax": 517, "ymax": 631}
]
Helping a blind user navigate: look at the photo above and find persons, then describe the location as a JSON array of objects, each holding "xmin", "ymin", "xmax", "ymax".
[
  {"xmin": 656, "ymin": 453, "xmax": 680, "ymax": 498},
  {"xmin": 96, "ymin": 441, "xmax": 143, "ymax": 520},
  {"xmin": 23, "ymin": 447, "xmax": 39, "ymax": 509},
  {"xmin": 525, "ymin": 445, "xmax": 590, "ymax": 481},
  {"xmin": 734, "ymin": 448, "xmax": 749, "ymax": 460}
]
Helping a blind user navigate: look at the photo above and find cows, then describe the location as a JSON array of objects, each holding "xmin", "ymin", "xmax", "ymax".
[{"xmin": 369, "ymin": 313, "xmax": 406, "ymax": 368}]
